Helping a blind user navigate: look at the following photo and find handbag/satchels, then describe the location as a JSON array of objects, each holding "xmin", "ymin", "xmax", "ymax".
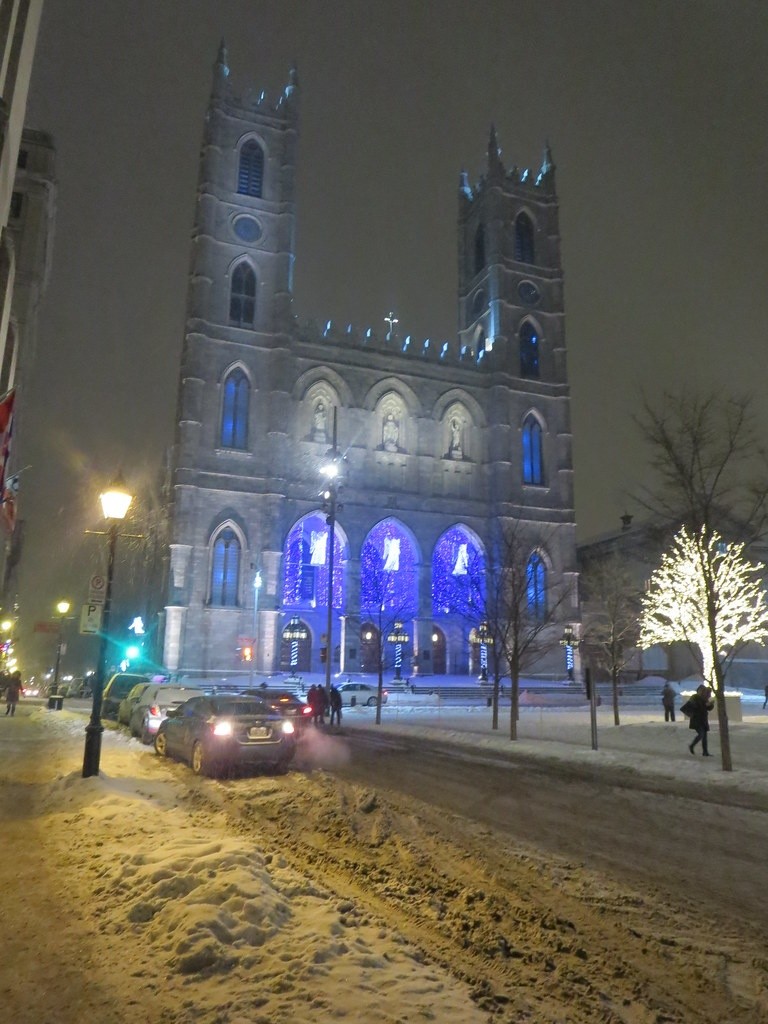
[
  {"xmin": 680, "ymin": 700, "xmax": 693, "ymax": 716},
  {"xmin": 339, "ymin": 709, "xmax": 342, "ymax": 719}
]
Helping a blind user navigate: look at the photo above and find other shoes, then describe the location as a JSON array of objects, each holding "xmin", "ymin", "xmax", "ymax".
[
  {"xmin": 689, "ymin": 745, "xmax": 695, "ymax": 755},
  {"xmin": 703, "ymin": 752, "xmax": 712, "ymax": 756}
]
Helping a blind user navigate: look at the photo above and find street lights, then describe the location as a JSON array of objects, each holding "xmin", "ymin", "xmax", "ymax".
[
  {"xmin": 48, "ymin": 594, "xmax": 70, "ymax": 696},
  {"xmin": 321, "ymin": 404, "xmax": 343, "ymax": 717},
  {"xmin": 79, "ymin": 469, "xmax": 133, "ymax": 776}
]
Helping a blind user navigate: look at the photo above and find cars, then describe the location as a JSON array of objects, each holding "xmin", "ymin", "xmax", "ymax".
[
  {"xmin": 153, "ymin": 692, "xmax": 296, "ymax": 776},
  {"xmin": 331, "ymin": 681, "xmax": 388, "ymax": 708},
  {"xmin": 117, "ymin": 682, "xmax": 206, "ymax": 744},
  {"xmin": 236, "ymin": 687, "xmax": 313, "ymax": 726}
]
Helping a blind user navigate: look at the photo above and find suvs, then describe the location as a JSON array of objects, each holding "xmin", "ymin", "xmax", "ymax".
[{"xmin": 98, "ymin": 672, "xmax": 153, "ymax": 718}]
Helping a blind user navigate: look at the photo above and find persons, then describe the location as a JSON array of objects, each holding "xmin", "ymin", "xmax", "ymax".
[
  {"xmin": 661, "ymin": 683, "xmax": 676, "ymax": 722},
  {"xmin": 687, "ymin": 684, "xmax": 716, "ymax": 756},
  {"xmin": 0, "ymin": 671, "xmax": 26, "ymax": 718},
  {"xmin": 308, "ymin": 683, "xmax": 343, "ymax": 728},
  {"xmin": 762, "ymin": 685, "xmax": 768, "ymax": 709}
]
